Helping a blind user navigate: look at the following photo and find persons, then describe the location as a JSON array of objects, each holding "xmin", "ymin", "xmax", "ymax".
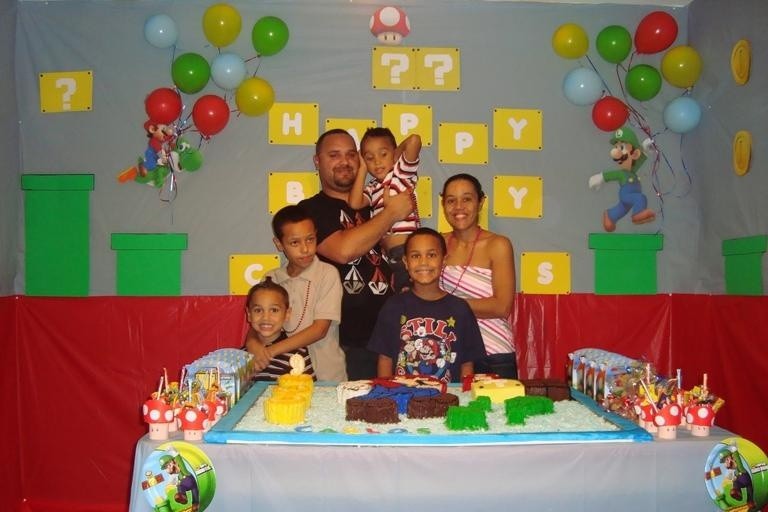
[
  {"xmin": 246, "ymin": 206, "xmax": 348, "ymax": 384},
  {"xmin": 243, "ymin": 280, "xmax": 318, "ymax": 385},
  {"xmin": 297, "ymin": 128, "xmax": 417, "ymax": 381},
  {"xmin": 439, "ymin": 173, "xmax": 517, "ymax": 380},
  {"xmin": 366, "ymin": 227, "xmax": 487, "ymax": 384},
  {"xmin": 348, "ymin": 128, "xmax": 422, "ymax": 295}
]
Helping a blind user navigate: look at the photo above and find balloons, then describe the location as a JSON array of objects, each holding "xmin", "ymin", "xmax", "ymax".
[
  {"xmin": 591, "ymin": 97, "xmax": 629, "ymax": 131},
  {"xmin": 211, "ymin": 51, "xmax": 246, "ymax": 99},
  {"xmin": 553, "ymin": 23, "xmax": 590, "ymax": 59},
  {"xmin": 203, "ymin": 4, "xmax": 242, "ymax": 49},
  {"xmin": 143, "ymin": 15, "xmax": 178, "ymax": 49},
  {"xmin": 662, "ymin": 96, "xmax": 701, "ymax": 134},
  {"xmin": 624, "ymin": 64, "xmax": 663, "ymax": 102},
  {"xmin": 563, "ymin": 68, "xmax": 607, "ymax": 106},
  {"xmin": 144, "ymin": 86, "xmax": 183, "ymax": 125},
  {"xmin": 250, "ymin": 17, "xmax": 288, "ymax": 57},
  {"xmin": 636, "ymin": 11, "xmax": 679, "ymax": 54},
  {"xmin": 596, "ymin": 25, "xmax": 633, "ymax": 63},
  {"xmin": 235, "ymin": 78, "xmax": 274, "ymax": 117},
  {"xmin": 191, "ymin": 94, "xmax": 230, "ymax": 142},
  {"xmin": 663, "ymin": 45, "xmax": 703, "ymax": 91},
  {"xmin": 170, "ymin": 53, "xmax": 212, "ymax": 95}
]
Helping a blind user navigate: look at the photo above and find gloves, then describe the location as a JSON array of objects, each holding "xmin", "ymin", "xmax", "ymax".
[
  {"xmin": 588, "ymin": 171, "xmax": 605, "ymax": 191},
  {"xmin": 170, "ymin": 476, "xmax": 177, "ymax": 485},
  {"xmin": 730, "ymin": 440, "xmax": 736, "ymax": 451},
  {"xmin": 641, "ymin": 138, "xmax": 653, "ymax": 152},
  {"xmin": 168, "ymin": 446, "xmax": 179, "ymax": 457},
  {"xmin": 725, "ymin": 472, "xmax": 735, "ymax": 480}
]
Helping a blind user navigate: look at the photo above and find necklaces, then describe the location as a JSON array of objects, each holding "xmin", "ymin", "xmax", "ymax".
[
  {"xmin": 441, "ymin": 225, "xmax": 487, "ymax": 295},
  {"xmin": 280, "ymin": 280, "xmax": 312, "ymax": 334}
]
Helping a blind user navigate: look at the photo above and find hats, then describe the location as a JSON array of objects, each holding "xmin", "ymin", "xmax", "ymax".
[
  {"xmin": 719, "ymin": 450, "xmax": 732, "ymax": 463},
  {"xmin": 159, "ymin": 456, "xmax": 175, "ymax": 470},
  {"xmin": 144, "ymin": 120, "xmax": 159, "ymax": 132},
  {"xmin": 609, "ymin": 128, "xmax": 639, "ymax": 148}
]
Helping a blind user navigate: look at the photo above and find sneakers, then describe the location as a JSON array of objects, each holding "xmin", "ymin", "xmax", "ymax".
[
  {"xmin": 730, "ymin": 489, "xmax": 743, "ymax": 501},
  {"xmin": 139, "ymin": 162, "xmax": 147, "ymax": 177},
  {"xmin": 192, "ymin": 504, "xmax": 200, "ymax": 511},
  {"xmin": 632, "ymin": 209, "xmax": 655, "ymax": 224},
  {"xmin": 604, "ymin": 211, "xmax": 617, "ymax": 233},
  {"xmin": 175, "ymin": 494, "xmax": 187, "ymax": 504},
  {"xmin": 748, "ymin": 502, "xmax": 754, "ymax": 509}
]
